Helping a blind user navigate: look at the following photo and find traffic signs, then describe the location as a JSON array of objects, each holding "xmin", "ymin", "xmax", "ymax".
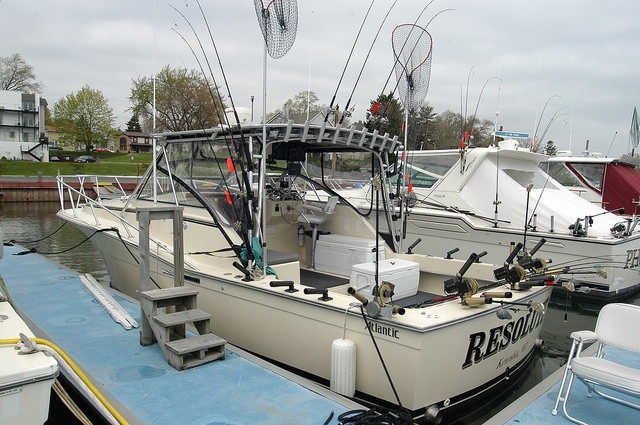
[{"xmin": 495, "ymin": 131, "xmax": 529, "ymax": 138}]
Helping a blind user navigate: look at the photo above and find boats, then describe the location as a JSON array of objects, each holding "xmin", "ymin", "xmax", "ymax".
[
  {"xmin": 52, "ymin": 120, "xmax": 561, "ymax": 424},
  {"xmin": 540, "ymin": 144, "xmax": 639, "ymax": 222},
  {"xmin": 1, "ymin": 279, "xmax": 61, "ymax": 424},
  {"xmin": 226, "ymin": 110, "xmax": 640, "ymax": 305}
]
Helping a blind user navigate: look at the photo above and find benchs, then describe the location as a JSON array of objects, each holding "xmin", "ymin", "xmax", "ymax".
[{"xmin": 267, "ymin": 248, "xmax": 300, "ymax": 283}]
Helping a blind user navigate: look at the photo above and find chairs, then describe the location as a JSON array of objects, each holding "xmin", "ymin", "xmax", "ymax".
[{"xmin": 551, "ymin": 303, "xmax": 640, "ymax": 424}]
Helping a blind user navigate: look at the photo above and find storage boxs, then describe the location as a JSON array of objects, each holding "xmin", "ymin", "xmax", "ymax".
[
  {"xmin": 350, "ymin": 259, "xmax": 420, "ymax": 302},
  {"xmin": 314, "ymin": 234, "xmax": 385, "ymax": 278}
]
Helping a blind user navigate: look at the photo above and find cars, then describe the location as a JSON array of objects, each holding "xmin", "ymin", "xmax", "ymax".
[
  {"xmin": 96, "ymin": 148, "xmax": 113, "ymax": 153},
  {"xmin": 75, "ymin": 155, "xmax": 96, "ymax": 163}
]
